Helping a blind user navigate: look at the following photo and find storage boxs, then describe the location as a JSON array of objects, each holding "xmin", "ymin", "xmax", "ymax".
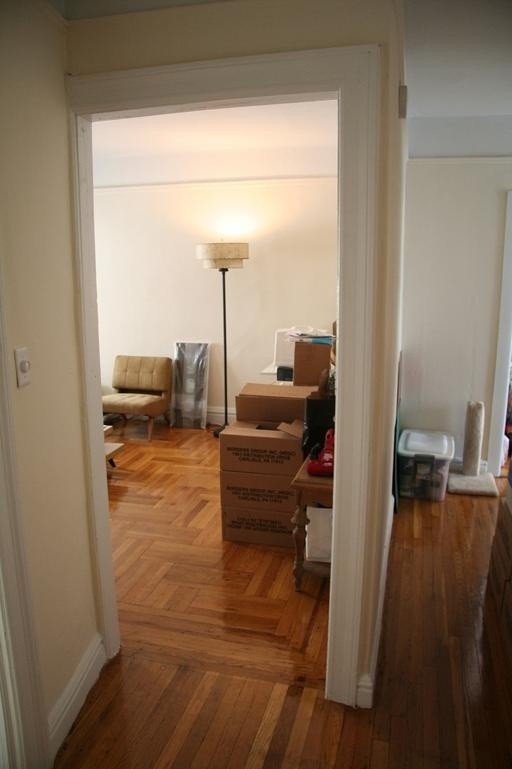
[
  {"xmin": 397, "ymin": 427, "xmax": 456, "ymax": 502},
  {"xmin": 217, "ymin": 342, "xmax": 331, "ymax": 548}
]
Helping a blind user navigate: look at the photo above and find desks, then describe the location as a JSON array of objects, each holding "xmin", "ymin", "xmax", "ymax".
[{"xmin": 288, "ymin": 453, "xmax": 333, "ymax": 594}]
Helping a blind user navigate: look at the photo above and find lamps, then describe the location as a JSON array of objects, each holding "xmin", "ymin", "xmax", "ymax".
[{"xmin": 196, "ymin": 242, "xmax": 248, "ymax": 438}]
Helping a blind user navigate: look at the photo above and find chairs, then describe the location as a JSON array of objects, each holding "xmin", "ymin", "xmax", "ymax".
[{"xmin": 102, "ymin": 356, "xmax": 173, "ymax": 442}]
